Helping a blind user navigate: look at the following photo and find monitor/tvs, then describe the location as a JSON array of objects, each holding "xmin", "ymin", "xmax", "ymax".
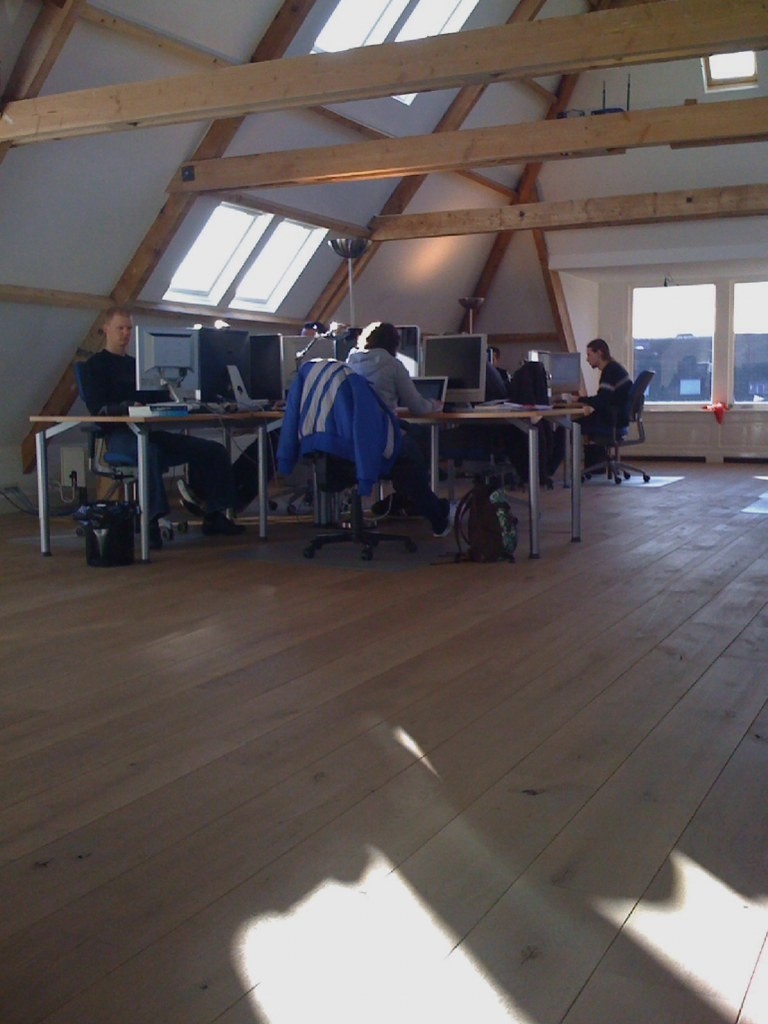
[{"xmin": 134, "ymin": 325, "xmax": 582, "ymax": 408}]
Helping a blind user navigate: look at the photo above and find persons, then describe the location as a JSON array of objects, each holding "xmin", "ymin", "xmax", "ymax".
[
  {"xmin": 302, "ymin": 322, "xmax": 325, "ymax": 337},
  {"xmin": 344, "ymin": 322, "xmax": 456, "ymax": 538},
  {"xmin": 522, "ymin": 338, "xmax": 633, "ymax": 486},
  {"xmin": 492, "ymin": 347, "xmax": 512, "ymax": 397},
  {"xmin": 80, "ymin": 306, "xmax": 246, "ymax": 550}
]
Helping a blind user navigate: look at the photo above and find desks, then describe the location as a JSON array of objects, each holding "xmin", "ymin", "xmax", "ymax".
[{"xmin": 26, "ymin": 405, "xmax": 591, "ymax": 564}]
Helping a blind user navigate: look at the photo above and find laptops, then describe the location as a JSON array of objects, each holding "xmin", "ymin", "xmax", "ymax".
[{"xmin": 398, "ymin": 376, "xmax": 447, "ymax": 412}]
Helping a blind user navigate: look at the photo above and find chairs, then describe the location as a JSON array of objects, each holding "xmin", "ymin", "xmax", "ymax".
[
  {"xmin": 71, "ymin": 360, "xmax": 141, "ymax": 538},
  {"xmin": 277, "ymin": 362, "xmax": 417, "ymax": 559},
  {"xmin": 580, "ymin": 369, "xmax": 656, "ymax": 486}
]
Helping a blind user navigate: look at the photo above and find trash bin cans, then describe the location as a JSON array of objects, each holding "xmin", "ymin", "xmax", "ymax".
[{"xmin": 83, "ymin": 501, "xmax": 136, "ymax": 567}]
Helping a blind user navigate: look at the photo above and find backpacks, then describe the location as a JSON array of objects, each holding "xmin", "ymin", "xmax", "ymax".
[{"xmin": 455, "ymin": 484, "xmax": 517, "ymax": 562}]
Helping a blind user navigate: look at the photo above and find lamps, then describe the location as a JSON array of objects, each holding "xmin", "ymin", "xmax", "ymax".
[
  {"xmin": 326, "ymin": 237, "xmax": 373, "ymax": 326},
  {"xmin": 459, "ymin": 296, "xmax": 486, "ymax": 331}
]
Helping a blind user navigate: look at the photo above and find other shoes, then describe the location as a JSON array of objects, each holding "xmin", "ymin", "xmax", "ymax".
[
  {"xmin": 372, "ymin": 495, "xmax": 426, "ymax": 520},
  {"xmin": 432, "ymin": 498, "xmax": 455, "ymax": 538},
  {"xmin": 202, "ymin": 516, "xmax": 245, "ymax": 535},
  {"xmin": 178, "ymin": 479, "xmax": 208, "ymax": 519}
]
